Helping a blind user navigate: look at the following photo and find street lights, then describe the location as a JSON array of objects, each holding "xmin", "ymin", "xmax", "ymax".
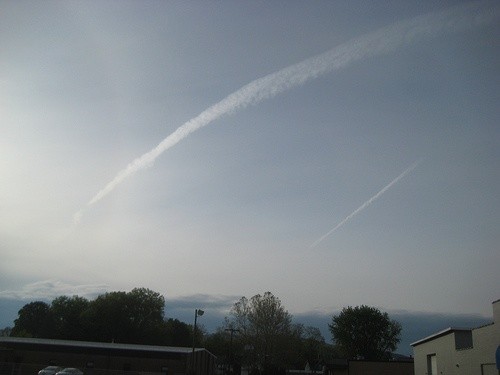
[{"xmin": 192, "ymin": 309, "xmax": 205, "ymax": 375}]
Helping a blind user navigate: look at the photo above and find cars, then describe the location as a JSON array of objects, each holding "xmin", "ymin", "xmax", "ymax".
[
  {"xmin": 38, "ymin": 366, "xmax": 62, "ymax": 375},
  {"xmin": 55, "ymin": 368, "xmax": 83, "ymax": 375}
]
